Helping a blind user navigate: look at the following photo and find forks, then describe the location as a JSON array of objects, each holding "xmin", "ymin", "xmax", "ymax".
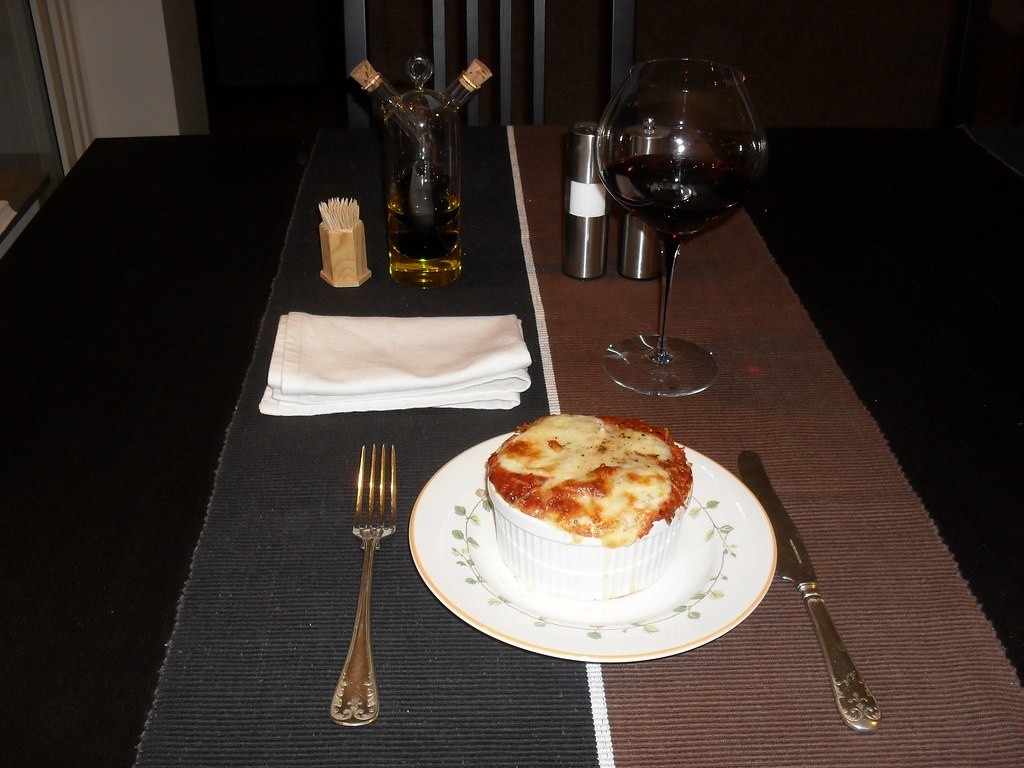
[{"xmin": 330, "ymin": 445, "xmax": 396, "ymax": 726}]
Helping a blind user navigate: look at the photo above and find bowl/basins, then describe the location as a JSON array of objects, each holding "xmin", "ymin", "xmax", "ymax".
[{"xmin": 487, "ymin": 462, "xmax": 693, "ymax": 602}]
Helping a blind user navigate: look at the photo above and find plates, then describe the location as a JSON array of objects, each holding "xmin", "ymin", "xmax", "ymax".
[{"xmin": 409, "ymin": 433, "xmax": 778, "ymax": 664}]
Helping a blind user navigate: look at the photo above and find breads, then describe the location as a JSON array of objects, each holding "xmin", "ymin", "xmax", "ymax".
[{"xmin": 487, "ymin": 414, "xmax": 691, "ymax": 549}]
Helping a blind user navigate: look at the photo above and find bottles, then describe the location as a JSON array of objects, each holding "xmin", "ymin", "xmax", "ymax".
[
  {"xmin": 563, "ymin": 121, "xmax": 608, "ymax": 278},
  {"xmin": 349, "ymin": 58, "xmax": 493, "ymax": 285},
  {"xmin": 618, "ymin": 117, "xmax": 671, "ymax": 281}
]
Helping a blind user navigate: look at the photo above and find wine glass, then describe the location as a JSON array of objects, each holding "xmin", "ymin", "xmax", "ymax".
[{"xmin": 593, "ymin": 58, "xmax": 759, "ymax": 396}]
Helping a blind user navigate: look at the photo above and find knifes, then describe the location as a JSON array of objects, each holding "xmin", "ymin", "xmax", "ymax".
[{"xmin": 738, "ymin": 450, "xmax": 882, "ymax": 734}]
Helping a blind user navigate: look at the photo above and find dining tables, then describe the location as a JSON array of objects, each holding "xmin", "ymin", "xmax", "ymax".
[{"xmin": 0, "ymin": 121, "xmax": 1024, "ymax": 768}]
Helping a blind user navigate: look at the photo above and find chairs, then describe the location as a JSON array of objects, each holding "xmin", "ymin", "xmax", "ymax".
[{"xmin": 343, "ymin": 0, "xmax": 636, "ymax": 129}]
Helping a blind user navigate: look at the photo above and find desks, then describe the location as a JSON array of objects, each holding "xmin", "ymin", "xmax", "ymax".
[{"xmin": 925, "ymin": 0, "xmax": 1024, "ymax": 187}]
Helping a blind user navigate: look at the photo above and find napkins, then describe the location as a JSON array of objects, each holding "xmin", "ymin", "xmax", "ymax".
[{"xmin": 258, "ymin": 310, "xmax": 534, "ymax": 416}]
